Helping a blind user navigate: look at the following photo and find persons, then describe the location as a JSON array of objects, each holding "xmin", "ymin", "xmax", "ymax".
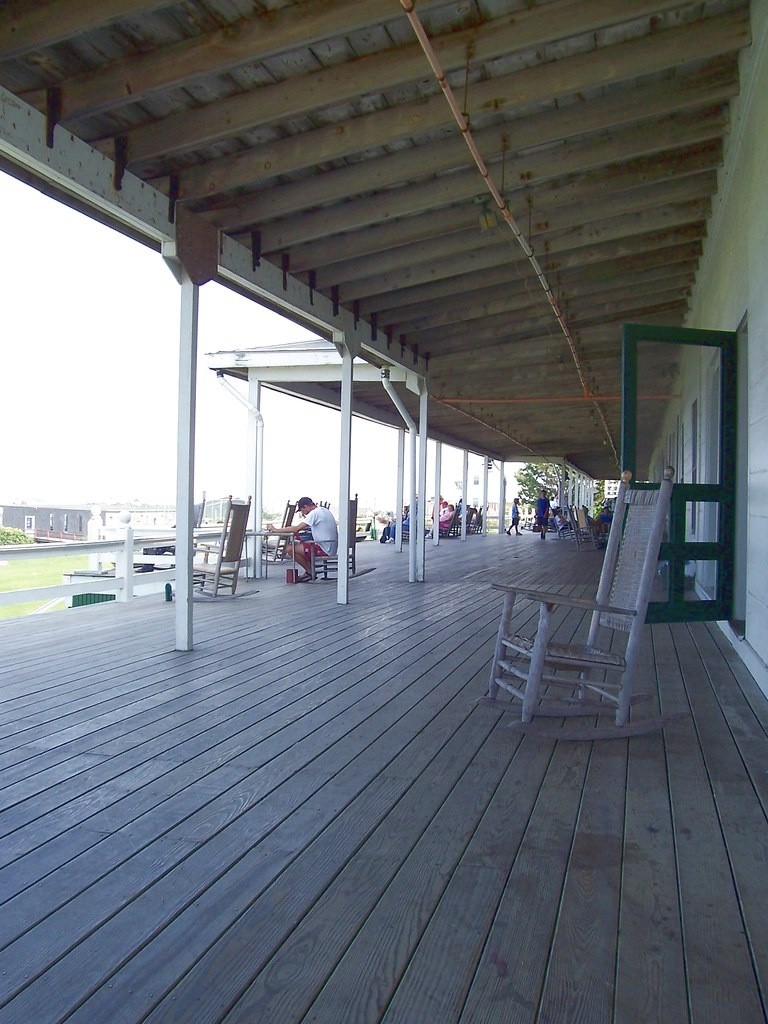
[
  {"xmin": 583, "ymin": 506, "xmax": 612, "ymax": 527},
  {"xmin": 425, "ymin": 494, "xmax": 478, "ymax": 539},
  {"xmin": 384, "ymin": 505, "xmax": 410, "ymax": 544},
  {"xmin": 554, "ymin": 506, "xmax": 572, "ymax": 530},
  {"xmin": 534, "ymin": 489, "xmax": 551, "ymax": 540},
  {"xmin": 506, "ymin": 498, "xmax": 523, "ymax": 536},
  {"xmin": 266, "ymin": 496, "xmax": 338, "ymax": 582}
]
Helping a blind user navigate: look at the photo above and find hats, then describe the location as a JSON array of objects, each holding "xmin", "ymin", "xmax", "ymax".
[
  {"xmin": 295, "ymin": 497, "xmax": 314, "ymax": 513},
  {"xmin": 441, "ymin": 501, "xmax": 448, "ymax": 506}
]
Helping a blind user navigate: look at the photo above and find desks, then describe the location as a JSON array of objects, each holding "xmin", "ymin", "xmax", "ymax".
[{"xmin": 243, "ymin": 532, "xmax": 312, "ymax": 584}]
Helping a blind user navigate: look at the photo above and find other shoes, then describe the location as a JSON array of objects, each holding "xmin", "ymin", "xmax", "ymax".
[
  {"xmin": 425, "ymin": 533, "xmax": 433, "ymax": 539},
  {"xmin": 507, "ymin": 531, "xmax": 511, "ymax": 535},
  {"xmin": 516, "ymin": 532, "xmax": 522, "ymax": 536}
]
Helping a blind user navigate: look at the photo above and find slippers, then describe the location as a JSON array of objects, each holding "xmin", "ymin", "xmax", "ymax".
[{"xmin": 298, "ymin": 572, "xmax": 318, "ymax": 582}]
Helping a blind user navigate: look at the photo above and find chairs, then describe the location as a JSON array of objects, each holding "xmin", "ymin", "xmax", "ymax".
[
  {"xmin": 402, "ymin": 529, "xmax": 409, "ymax": 541},
  {"xmin": 441, "ymin": 467, "xmax": 682, "ymax": 738},
  {"xmin": 193, "ymin": 493, "xmax": 377, "ymax": 602}
]
